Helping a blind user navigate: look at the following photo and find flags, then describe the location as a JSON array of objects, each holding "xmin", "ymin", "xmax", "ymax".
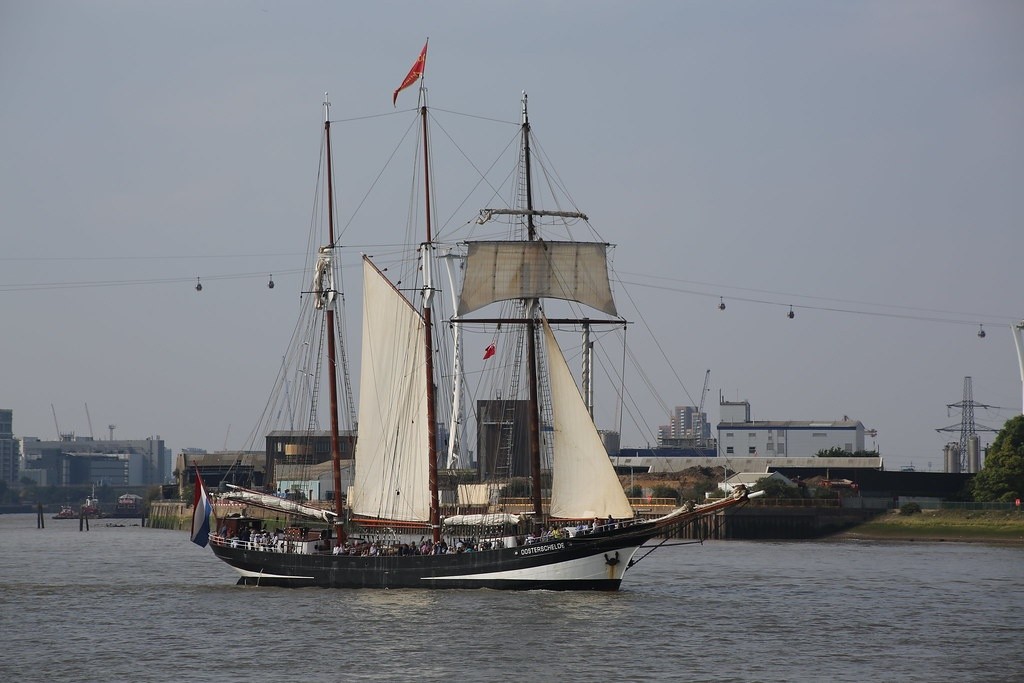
[
  {"xmin": 483, "ymin": 342, "xmax": 495, "ymax": 360},
  {"xmin": 190, "ymin": 471, "xmax": 213, "ymax": 548},
  {"xmin": 393, "ymin": 40, "xmax": 428, "ymax": 108}
]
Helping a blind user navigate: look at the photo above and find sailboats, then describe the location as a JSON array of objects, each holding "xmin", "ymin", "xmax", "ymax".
[{"xmin": 205, "ymin": 36, "xmax": 772, "ymax": 589}]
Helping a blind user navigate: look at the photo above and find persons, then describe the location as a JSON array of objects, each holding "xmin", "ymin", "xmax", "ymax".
[{"xmin": 221, "ymin": 515, "xmax": 615, "ymax": 557}]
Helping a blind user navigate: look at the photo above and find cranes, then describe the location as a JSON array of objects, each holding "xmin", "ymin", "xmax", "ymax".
[{"xmin": 694, "ymin": 369, "xmax": 712, "ymax": 436}]
